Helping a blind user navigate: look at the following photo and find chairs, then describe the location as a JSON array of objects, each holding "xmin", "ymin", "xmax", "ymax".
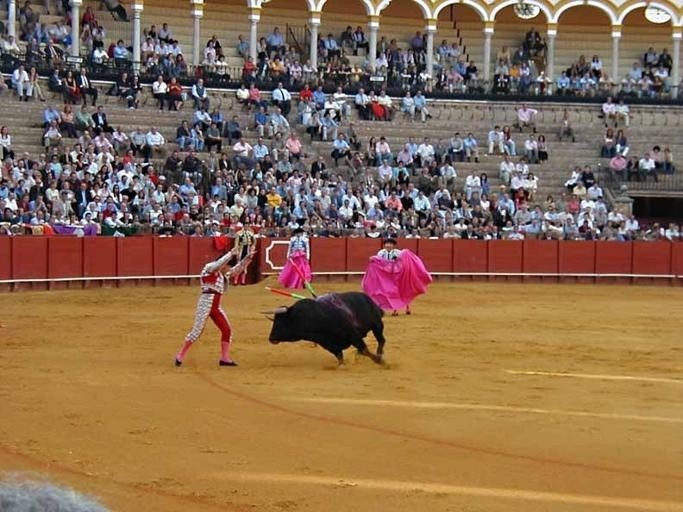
[{"xmin": 1, "ymin": 75, "xmax": 683, "ymax": 203}]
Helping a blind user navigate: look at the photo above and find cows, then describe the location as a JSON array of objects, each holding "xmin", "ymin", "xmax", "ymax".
[{"xmin": 259, "ymin": 290, "xmax": 386, "ymax": 369}]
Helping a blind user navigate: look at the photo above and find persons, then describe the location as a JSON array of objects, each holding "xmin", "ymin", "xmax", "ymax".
[
  {"xmin": 285, "ymin": 226, "xmax": 310, "ymax": 290},
  {"xmin": 172, "ymin": 244, "xmax": 258, "ymax": 367},
  {"xmin": 375, "ymin": 236, "xmax": 412, "ymax": 318},
  {"xmin": 232, "ymin": 221, "xmax": 257, "ymax": 286},
  {"xmin": 2, "ymin": 4, "xmax": 679, "ymax": 241}
]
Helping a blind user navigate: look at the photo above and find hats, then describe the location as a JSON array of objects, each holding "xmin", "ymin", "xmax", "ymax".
[
  {"xmin": 294, "ymin": 227, "xmax": 304, "ymax": 234},
  {"xmin": 384, "ymin": 237, "xmax": 397, "ymax": 245}
]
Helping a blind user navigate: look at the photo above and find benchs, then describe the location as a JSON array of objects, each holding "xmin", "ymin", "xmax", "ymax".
[{"xmin": 1, "ymin": 0, "xmax": 683, "ymax": 96}]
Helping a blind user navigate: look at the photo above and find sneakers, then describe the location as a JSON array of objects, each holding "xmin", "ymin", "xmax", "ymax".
[
  {"xmin": 406, "ymin": 311, "xmax": 410, "ymax": 315},
  {"xmin": 220, "ymin": 358, "xmax": 237, "ymax": 366},
  {"xmin": 392, "ymin": 312, "xmax": 398, "ymax": 316},
  {"xmin": 175, "ymin": 357, "xmax": 182, "ymax": 365}
]
